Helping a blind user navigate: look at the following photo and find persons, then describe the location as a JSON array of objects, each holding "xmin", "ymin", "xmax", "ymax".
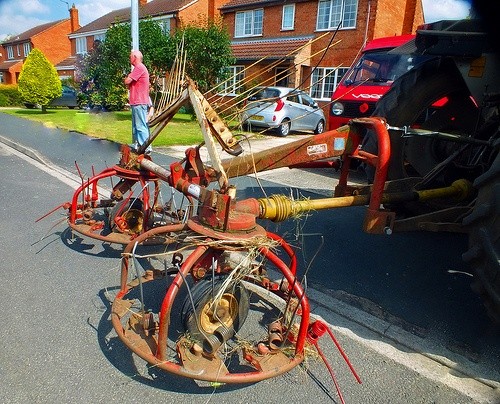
[{"xmin": 124, "ymin": 49, "xmax": 153, "ymax": 156}]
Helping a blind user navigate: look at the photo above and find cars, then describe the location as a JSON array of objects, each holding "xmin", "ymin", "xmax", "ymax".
[
  {"xmin": 241, "ymin": 86, "xmax": 326, "ymax": 137},
  {"xmin": 24, "ymin": 86, "xmax": 80, "ymax": 109}
]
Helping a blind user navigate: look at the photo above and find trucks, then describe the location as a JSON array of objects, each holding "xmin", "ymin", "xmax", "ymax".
[{"xmin": 329, "ymin": 34, "xmax": 448, "ymax": 169}]
